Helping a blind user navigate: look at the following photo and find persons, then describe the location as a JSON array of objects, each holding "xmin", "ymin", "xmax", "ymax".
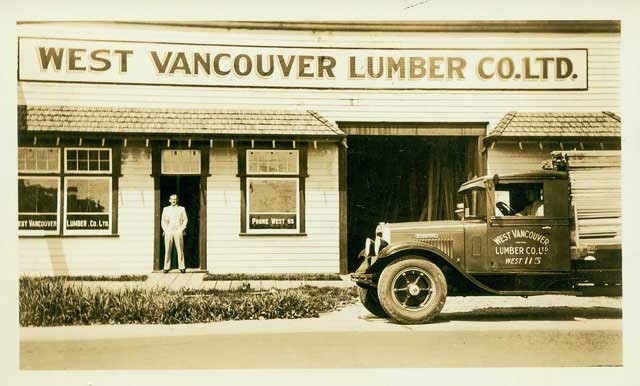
[
  {"xmin": 160, "ymin": 193, "xmax": 189, "ymax": 273},
  {"xmin": 516, "ymin": 184, "xmax": 544, "ymax": 217}
]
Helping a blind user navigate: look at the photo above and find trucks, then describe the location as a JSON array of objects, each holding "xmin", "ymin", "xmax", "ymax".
[{"xmin": 348, "ymin": 150, "xmax": 622, "ymax": 323}]
